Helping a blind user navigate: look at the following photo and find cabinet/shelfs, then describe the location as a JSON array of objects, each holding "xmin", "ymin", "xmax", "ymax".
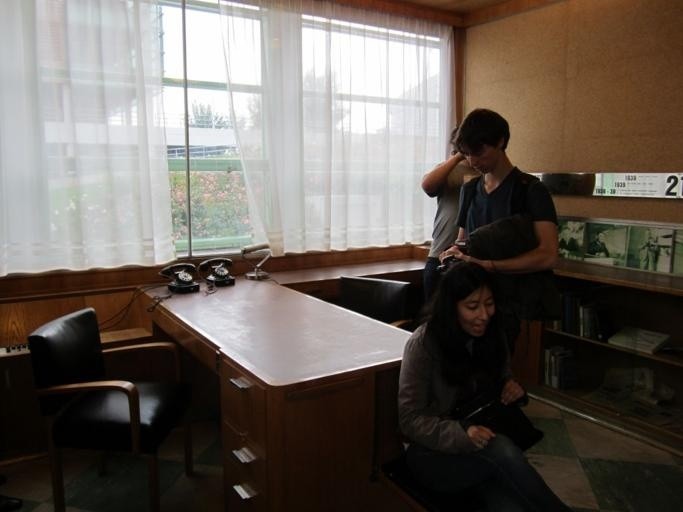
[{"xmin": 511, "ymin": 259, "xmax": 683, "ymax": 457}]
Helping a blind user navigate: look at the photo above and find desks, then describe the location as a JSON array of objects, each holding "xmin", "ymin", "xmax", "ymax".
[
  {"xmin": 267, "ymin": 257, "xmax": 426, "ymax": 306},
  {"xmin": 137, "ymin": 275, "xmax": 412, "ymax": 512}
]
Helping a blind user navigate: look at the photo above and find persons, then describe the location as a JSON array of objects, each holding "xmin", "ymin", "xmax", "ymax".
[
  {"xmin": 394, "ymin": 257, "xmax": 575, "ymax": 512},
  {"xmin": 436, "ymin": 108, "xmax": 560, "ymax": 378},
  {"xmin": 558, "ymin": 226, "xmax": 578, "ymax": 255},
  {"xmin": 586, "ymin": 230, "xmax": 608, "ymax": 257},
  {"xmin": 636, "ymin": 227, "xmax": 657, "ymax": 271},
  {"xmin": 420, "ymin": 126, "xmax": 480, "ymax": 304}
]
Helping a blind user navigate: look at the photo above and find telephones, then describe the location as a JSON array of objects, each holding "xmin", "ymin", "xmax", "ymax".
[
  {"xmin": 198, "ymin": 257, "xmax": 235, "ymax": 287},
  {"xmin": 160, "ymin": 263, "xmax": 199, "ymax": 293}
]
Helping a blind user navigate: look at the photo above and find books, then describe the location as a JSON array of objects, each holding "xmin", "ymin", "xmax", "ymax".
[{"xmin": 543, "ymin": 282, "xmax": 670, "ymax": 393}]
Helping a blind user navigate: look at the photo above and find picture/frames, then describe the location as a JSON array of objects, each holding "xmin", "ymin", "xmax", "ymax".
[{"xmin": 556, "ymin": 215, "xmax": 683, "ymax": 278}]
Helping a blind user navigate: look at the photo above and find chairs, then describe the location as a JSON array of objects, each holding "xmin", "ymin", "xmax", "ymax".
[
  {"xmin": 342, "ymin": 277, "xmax": 415, "ymax": 327},
  {"xmin": 27, "ymin": 307, "xmax": 193, "ymax": 512},
  {"xmin": 370, "ymin": 366, "xmax": 488, "ymax": 512}
]
